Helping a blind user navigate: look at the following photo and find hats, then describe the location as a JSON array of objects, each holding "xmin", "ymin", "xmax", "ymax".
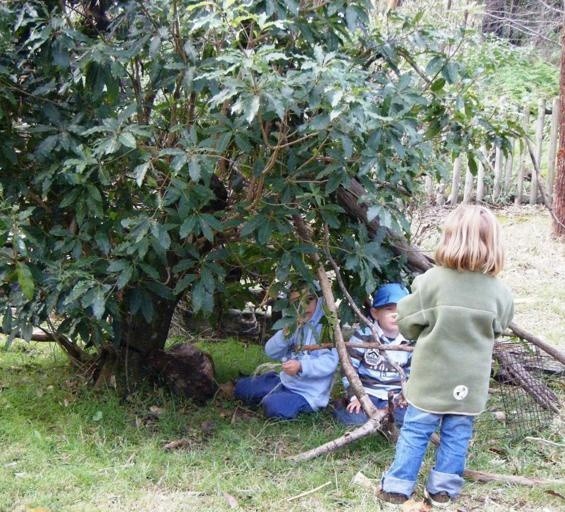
[{"xmin": 372, "ymin": 283, "xmax": 410, "ymax": 308}]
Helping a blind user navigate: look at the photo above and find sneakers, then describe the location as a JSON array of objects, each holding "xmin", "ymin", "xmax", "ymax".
[
  {"xmin": 376, "ymin": 487, "xmax": 407, "ymax": 506},
  {"xmin": 423, "ymin": 486, "xmax": 451, "ymax": 509},
  {"xmin": 334, "ymin": 396, "xmax": 348, "ymax": 410}
]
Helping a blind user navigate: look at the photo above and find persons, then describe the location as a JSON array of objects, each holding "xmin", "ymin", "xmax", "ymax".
[
  {"xmin": 332, "ymin": 283, "xmax": 418, "ymax": 430},
  {"xmin": 217, "ymin": 276, "xmax": 340, "ymax": 424},
  {"xmin": 370, "ymin": 203, "xmax": 517, "ymax": 509}
]
